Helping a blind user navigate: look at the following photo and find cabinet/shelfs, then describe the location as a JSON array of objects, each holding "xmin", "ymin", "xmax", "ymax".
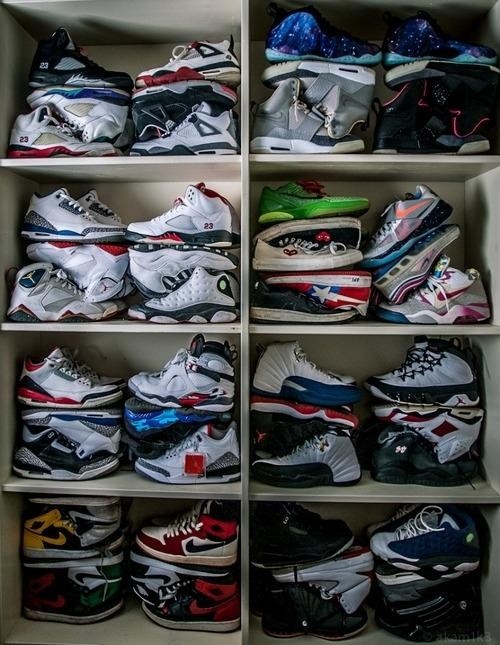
[
  {"xmin": 0, "ymin": 1, "xmax": 243, "ymax": 641},
  {"xmin": 245, "ymin": 0, "xmax": 500, "ymax": 645}
]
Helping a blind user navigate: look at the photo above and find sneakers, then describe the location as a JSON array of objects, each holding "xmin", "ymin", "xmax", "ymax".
[
  {"xmin": 248, "ymin": 175, "xmax": 491, "ymax": 325},
  {"xmin": 5, "ymin": 27, "xmax": 241, "ymax": 159},
  {"xmin": 9, "ymin": 332, "xmax": 241, "ymax": 486},
  {"xmin": 4, "ymin": 181, "xmax": 240, "ymax": 323},
  {"xmin": 19, "ymin": 494, "xmax": 240, "ymax": 634},
  {"xmin": 248, "ymin": 502, "xmax": 491, "ymax": 641},
  {"xmin": 248, "ymin": 334, "xmax": 487, "ymax": 490},
  {"xmin": 248, "ymin": 7, "xmax": 499, "ymax": 155}
]
